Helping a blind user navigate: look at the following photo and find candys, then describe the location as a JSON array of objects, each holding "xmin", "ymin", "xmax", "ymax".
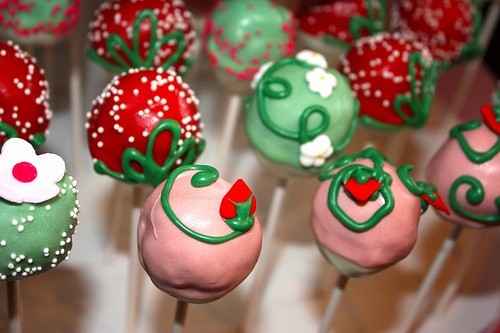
[{"xmin": 0, "ymin": 0, "xmax": 500, "ymax": 333}]
